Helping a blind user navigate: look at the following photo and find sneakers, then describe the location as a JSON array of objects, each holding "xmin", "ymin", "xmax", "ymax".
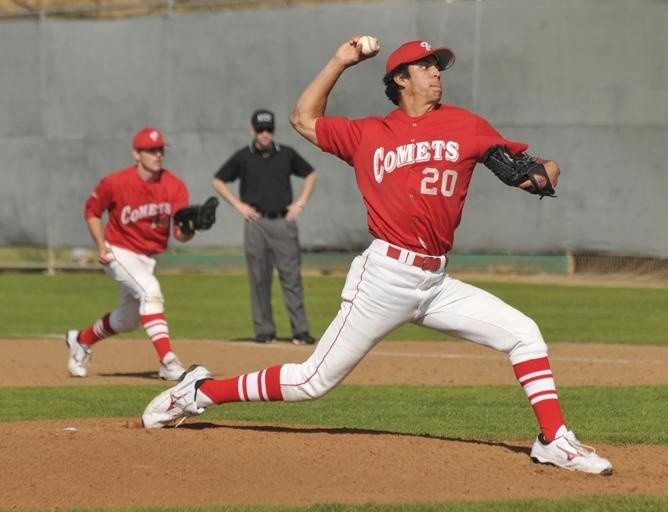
[
  {"xmin": 63, "ymin": 328, "xmax": 87, "ymax": 378},
  {"xmin": 141, "ymin": 364, "xmax": 209, "ymax": 430},
  {"xmin": 529, "ymin": 424, "xmax": 613, "ymax": 476},
  {"xmin": 158, "ymin": 354, "xmax": 185, "ymax": 380},
  {"xmin": 259, "ymin": 334, "xmax": 316, "ymax": 346}
]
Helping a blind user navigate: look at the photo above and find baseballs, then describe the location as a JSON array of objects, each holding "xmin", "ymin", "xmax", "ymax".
[{"xmin": 357, "ymin": 36, "xmax": 377, "ymax": 55}]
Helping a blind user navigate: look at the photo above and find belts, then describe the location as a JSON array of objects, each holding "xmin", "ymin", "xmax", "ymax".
[
  {"xmin": 385, "ymin": 246, "xmax": 449, "ymax": 273},
  {"xmin": 260, "ymin": 209, "xmax": 287, "ymax": 220}
]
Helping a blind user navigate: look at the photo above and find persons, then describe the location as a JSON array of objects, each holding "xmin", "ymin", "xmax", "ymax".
[
  {"xmin": 141, "ymin": 34, "xmax": 614, "ymax": 475},
  {"xmin": 211, "ymin": 109, "xmax": 318, "ymax": 345},
  {"xmin": 66, "ymin": 128, "xmax": 219, "ymax": 380}
]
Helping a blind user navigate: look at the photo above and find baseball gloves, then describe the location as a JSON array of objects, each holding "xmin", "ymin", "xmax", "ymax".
[
  {"xmin": 482, "ymin": 143, "xmax": 554, "ymax": 196},
  {"xmin": 174, "ymin": 196, "xmax": 219, "ymax": 234}
]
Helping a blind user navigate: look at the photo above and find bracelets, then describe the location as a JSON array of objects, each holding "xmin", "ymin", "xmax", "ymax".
[{"xmin": 295, "ymin": 199, "xmax": 306, "ymax": 208}]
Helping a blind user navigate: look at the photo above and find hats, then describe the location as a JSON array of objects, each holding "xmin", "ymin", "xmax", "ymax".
[
  {"xmin": 249, "ymin": 108, "xmax": 274, "ymax": 131},
  {"xmin": 129, "ymin": 128, "xmax": 169, "ymax": 150},
  {"xmin": 384, "ymin": 40, "xmax": 459, "ymax": 72}
]
